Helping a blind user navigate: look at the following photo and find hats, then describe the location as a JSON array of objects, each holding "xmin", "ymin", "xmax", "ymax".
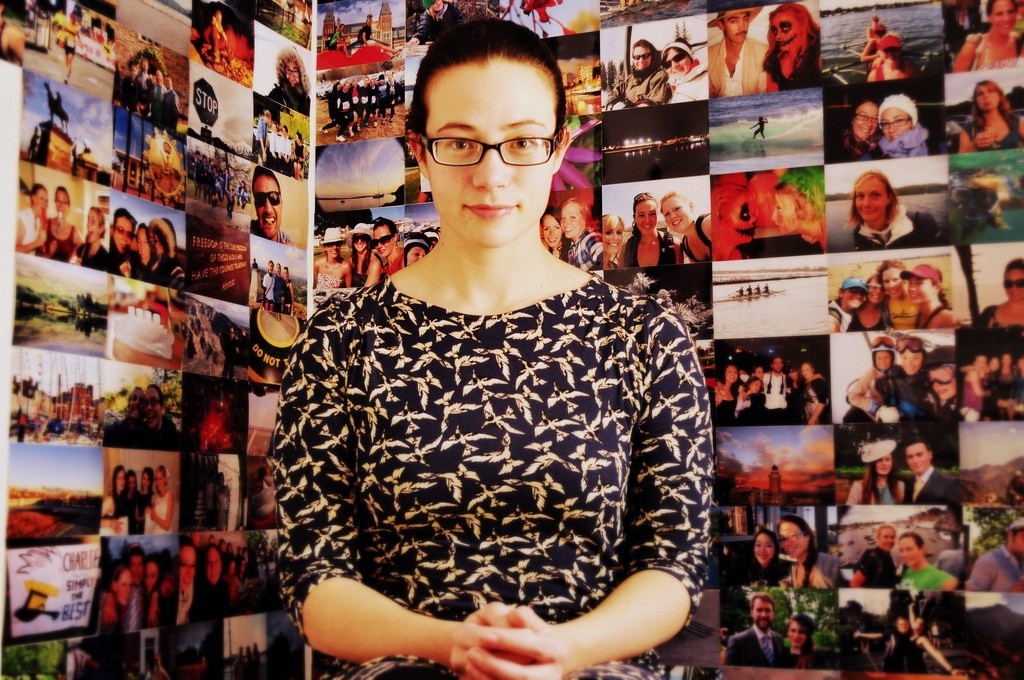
[
  {"xmin": 404, "ymin": 231, "xmax": 432, "ymax": 256},
  {"xmin": 423, "ymin": 228, "xmax": 439, "ymax": 240},
  {"xmin": 873, "ymin": 24, "xmax": 885, "ymax": 32},
  {"xmin": 346, "ymin": 223, "xmax": 373, "ymax": 248},
  {"xmin": 899, "ymin": 264, "xmax": 940, "ymax": 284},
  {"xmin": 877, "ymin": 34, "xmax": 902, "ymax": 51},
  {"xmin": 423, "ymin": 0, "xmax": 436, "ymax": 8},
  {"xmin": 860, "ymin": 439, "xmax": 896, "ymax": 462},
  {"xmin": 707, "ymin": 5, "xmax": 763, "ymax": 28},
  {"xmin": 878, "ymin": 93, "xmax": 917, "ymax": 126},
  {"xmin": 1008, "ymin": 517, "xmax": 1024, "ymax": 529},
  {"xmin": 321, "ymin": 228, "xmax": 344, "ymax": 245},
  {"xmin": 842, "ymin": 278, "xmax": 869, "ymax": 294}
]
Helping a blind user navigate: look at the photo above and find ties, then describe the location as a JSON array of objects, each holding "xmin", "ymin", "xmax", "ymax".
[
  {"xmin": 912, "ymin": 477, "xmax": 924, "ymax": 501},
  {"xmin": 760, "ymin": 636, "xmax": 773, "ymax": 664},
  {"xmin": 127, "ymin": 588, "xmax": 139, "ymax": 631}
]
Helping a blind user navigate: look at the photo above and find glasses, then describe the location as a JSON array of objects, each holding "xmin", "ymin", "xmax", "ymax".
[
  {"xmin": 926, "ymin": 365, "xmax": 956, "ymax": 385},
  {"xmin": 420, "ymin": 124, "xmax": 560, "ymax": 166},
  {"xmin": 947, "ymin": 186, "xmax": 999, "ymax": 211},
  {"xmin": 1003, "ymin": 278, "xmax": 1024, "ymax": 288},
  {"xmin": 780, "ymin": 530, "xmax": 805, "ymax": 545},
  {"xmin": 254, "ymin": 191, "xmax": 281, "ymax": 208},
  {"xmin": 661, "ymin": 51, "xmax": 687, "ymax": 71},
  {"xmin": 114, "ymin": 226, "xmax": 135, "ymax": 240},
  {"xmin": 633, "ymin": 192, "xmax": 654, "ymax": 200},
  {"xmin": 284, "ymin": 68, "xmax": 300, "ymax": 78},
  {"xmin": 370, "ymin": 233, "xmax": 394, "ymax": 249},
  {"xmin": 854, "ymin": 113, "xmax": 878, "ymax": 124},
  {"xmin": 879, "ymin": 117, "xmax": 912, "ymax": 130},
  {"xmin": 144, "ymin": 398, "xmax": 161, "ymax": 406},
  {"xmin": 352, "ymin": 235, "xmax": 370, "ymax": 244},
  {"xmin": 323, "ymin": 241, "xmax": 343, "ymax": 247},
  {"xmin": 632, "ymin": 52, "xmax": 652, "ymax": 60},
  {"xmin": 871, "ymin": 336, "xmax": 894, "ymax": 349},
  {"xmin": 896, "ymin": 336, "xmax": 924, "ymax": 354}
]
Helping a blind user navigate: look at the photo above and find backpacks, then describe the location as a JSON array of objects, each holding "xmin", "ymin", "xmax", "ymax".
[{"xmin": 325, "ymin": 33, "xmax": 335, "ymax": 47}]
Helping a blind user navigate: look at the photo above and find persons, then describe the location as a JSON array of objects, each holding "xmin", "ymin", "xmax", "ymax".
[
  {"xmin": 0, "ymin": 0, "xmax": 311, "ymax": 680},
  {"xmin": 313, "ymin": 1, "xmax": 1024, "ymax": 335},
  {"xmin": 267, "ymin": 13, "xmax": 718, "ymax": 680},
  {"xmin": 699, "ymin": 336, "xmax": 1023, "ymax": 679}
]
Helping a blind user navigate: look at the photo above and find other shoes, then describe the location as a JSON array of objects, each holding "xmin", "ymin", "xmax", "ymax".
[
  {"xmin": 335, "ymin": 136, "xmax": 345, "ymax": 142},
  {"xmin": 357, "ymin": 117, "xmax": 393, "ymax": 131},
  {"xmin": 319, "ymin": 128, "xmax": 328, "ymax": 133},
  {"xmin": 348, "ymin": 126, "xmax": 354, "ymax": 136}
]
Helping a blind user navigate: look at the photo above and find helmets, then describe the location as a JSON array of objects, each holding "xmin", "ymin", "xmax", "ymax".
[
  {"xmin": 922, "ymin": 346, "xmax": 957, "ymax": 368},
  {"xmin": 870, "ymin": 335, "xmax": 897, "ymax": 365}
]
